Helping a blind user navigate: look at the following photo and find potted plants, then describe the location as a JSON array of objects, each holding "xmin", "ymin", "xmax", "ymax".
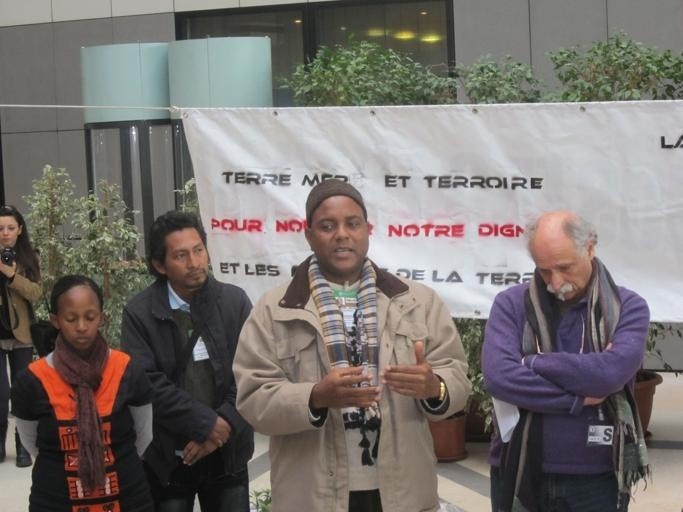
[
  {"xmin": 629, "ymin": 312, "xmax": 682, "ymax": 442},
  {"xmin": 423, "ymin": 311, "xmax": 493, "ymax": 463}
]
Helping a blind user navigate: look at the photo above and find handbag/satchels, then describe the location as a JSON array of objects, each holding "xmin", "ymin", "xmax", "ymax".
[{"xmin": 31, "ymin": 318, "xmax": 58, "ymax": 355}]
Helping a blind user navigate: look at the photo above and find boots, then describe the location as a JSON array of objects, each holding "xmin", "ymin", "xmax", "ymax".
[{"xmin": 14, "ymin": 431, "xmax": 32, "ymax": 467}]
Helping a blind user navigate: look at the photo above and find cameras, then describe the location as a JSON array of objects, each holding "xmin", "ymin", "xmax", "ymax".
[{"xmin": 0, "ymin": 247, "xmax": 16, "ymax": 266}]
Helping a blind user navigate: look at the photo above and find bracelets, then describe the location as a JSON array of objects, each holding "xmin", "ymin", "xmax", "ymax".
[{"xmin": 438, "ymin": 380, "xmax": 446, "ymax": 404}]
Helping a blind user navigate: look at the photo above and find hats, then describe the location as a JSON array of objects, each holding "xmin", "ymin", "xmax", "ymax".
[{"xmin": 305, "ymin": 178, "xmax": 367, "ymax": 226}]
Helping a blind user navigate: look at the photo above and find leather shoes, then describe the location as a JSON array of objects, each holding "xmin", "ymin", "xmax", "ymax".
[{"xmin": 0, "ymin": 424, "xmax": 8, "ymax": 462}]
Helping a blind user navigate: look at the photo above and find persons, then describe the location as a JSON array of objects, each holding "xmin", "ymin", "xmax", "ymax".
[
  {"xmin": 122, "ymin": 209, "xmax": 253, "ymax": 512},
  {"xmin": 10, "ymin": 274, "xmax": 154, "ymax": 512},
  {"xmin": 231, "ymin": 177, "xmax": 472, "ymax": 512},
  {"xmin": 0, "ymin": 204, "xmax": 43, "ymax": 468},
  {"xmin": 481, "ymin": 209, "xmax": 652, "ymax": 512}
]
